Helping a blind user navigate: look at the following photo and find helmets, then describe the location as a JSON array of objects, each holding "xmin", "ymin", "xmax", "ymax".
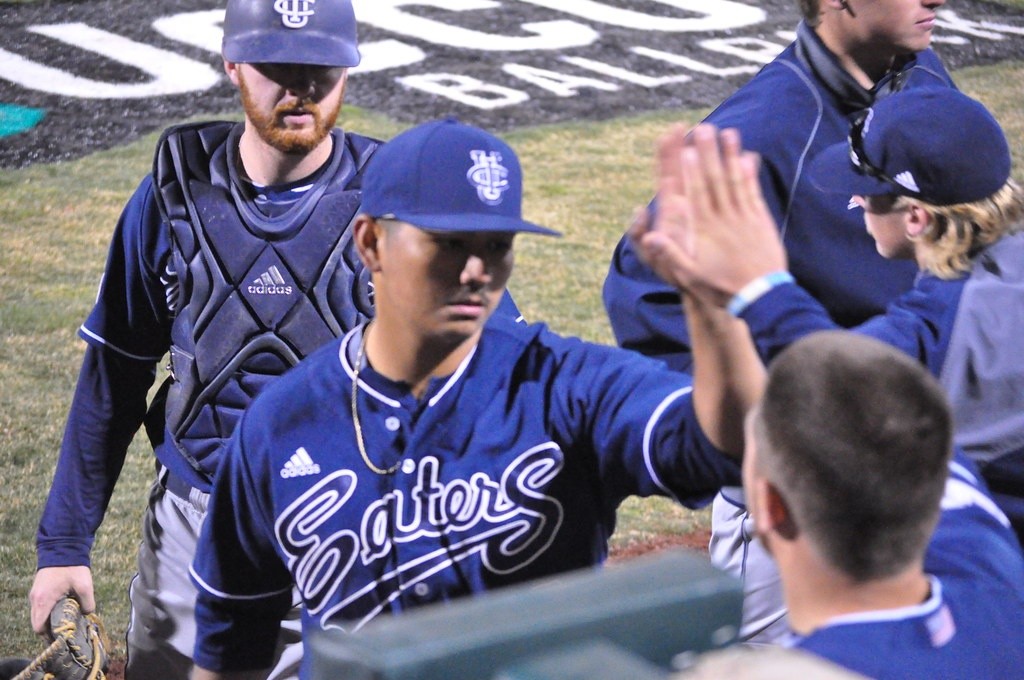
[{"xmin": 222, "ymin": 0, "xmax": 361, "ymax": 67}]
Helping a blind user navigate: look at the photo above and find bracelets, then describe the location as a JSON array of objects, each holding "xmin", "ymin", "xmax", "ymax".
[{"xmin": 725, "ymin": 271, "xmax": 796, "ymax": 319}]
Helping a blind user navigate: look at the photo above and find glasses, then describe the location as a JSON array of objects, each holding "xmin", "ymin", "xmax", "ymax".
[{"xmin": 845, "ymin": 109, "xmax": 884, "ymax": 177}]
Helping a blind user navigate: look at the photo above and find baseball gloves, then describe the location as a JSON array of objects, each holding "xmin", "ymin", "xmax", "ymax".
[{"xmin": 0, "ymin": 595, "xmax": 113, "ymax": 678}]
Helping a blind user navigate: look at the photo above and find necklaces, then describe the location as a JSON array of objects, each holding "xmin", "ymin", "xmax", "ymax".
[{"xmin": 352, "ymin": 318, "xmax": 404, "ymax": 476}]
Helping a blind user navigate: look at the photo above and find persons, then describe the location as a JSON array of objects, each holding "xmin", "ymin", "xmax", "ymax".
[
  {"xmin": 191, "ymin": 117, "xmax": 770, "ymax": 680},
  {"xmin": 601, "ymin": 0, "xmax": 1024, "ymax": 680},
  {"xmin": 28, "ymin": 1, "xmax": 526, "ymax": 680}
]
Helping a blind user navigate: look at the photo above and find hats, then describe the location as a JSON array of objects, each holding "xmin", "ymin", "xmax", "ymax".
[
  {"xmin": 806, "ymin": 84, "xmax": 1011, "ymax": 204},
  {"xmin": 360, "ymin": 118, "xmax": 564, "ymax": 238}
]
